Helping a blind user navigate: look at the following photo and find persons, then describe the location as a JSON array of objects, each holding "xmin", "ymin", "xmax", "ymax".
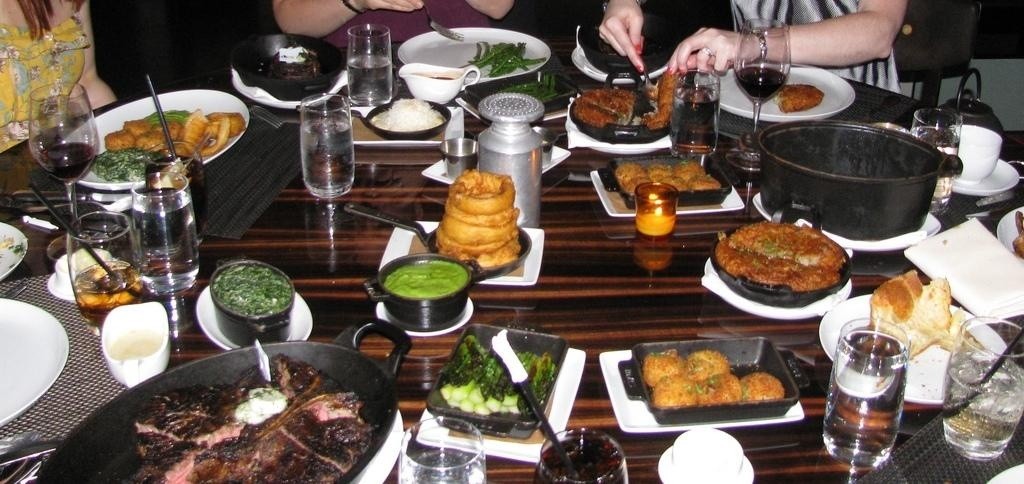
[
  {"xmin": 271, "ymin": 1, "xmax": 513, "ymax": 50},
  {"xmin": 1, "ymin": 0, "xmax": 117, "ymax": 155},
  {"xmin": 599, "ymin": 0, "xmax": 908, "ymax": 95}
]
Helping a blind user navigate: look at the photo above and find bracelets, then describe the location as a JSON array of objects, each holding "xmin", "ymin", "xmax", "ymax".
[
  {"xmin": 750, "ymin": 27, "xmax": 767, "ymax": 62},
  {"xmin": 342, "ymin": 0, "xmax": 365, "ymax": 15},
  {"xmin": 603, "ymin": 0, "xmax": 643, "ymax": 6}
]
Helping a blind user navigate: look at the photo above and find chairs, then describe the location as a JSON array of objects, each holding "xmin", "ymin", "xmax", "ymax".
[{"xmin": 892, "ymin": 0, "xmax": 982, "ymax": 107}]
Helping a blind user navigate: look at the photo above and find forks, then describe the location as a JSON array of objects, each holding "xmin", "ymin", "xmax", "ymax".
[
  {"xmin": 227, "ymin": 88, "xmax": 285, "ymax": 129},
  {"xmin": 421, "ymin": 3, "xmax": 465, "ymax": 42}
]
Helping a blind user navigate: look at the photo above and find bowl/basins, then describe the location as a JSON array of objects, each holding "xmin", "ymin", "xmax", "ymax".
[
  {"xmin": 102, "ymin": 301, "xmax": 170, "ymax": 389},
  {"xmin": 439, "ymin": 138, "xmax": 479, "ymax": 179},
  {"xmin": 533, "ymin": 125, "xmax": 557, "ymax": 171},
  {"xmin": 363, "ymin": 252, "xmax": 484, "ymax": 335},
  {"xmin": 397, "ymin": 61, "xmax": 481, "ymax": 102}
]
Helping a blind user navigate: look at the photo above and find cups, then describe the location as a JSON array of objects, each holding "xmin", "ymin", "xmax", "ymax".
[
  {"xmin": 299, "ymin": 93, "xmax": 355, "ymax": 199},
  {"xmin": 634, "ymin": 182, "xmax": 678, "ymax": 236},
  {"xmin": 66, "ymin": 211, "xmax": 144, "ymax": 336},
  {"xmin": 911, "ymin": 108, "xmax": 964, "ymax": 215},
  {"xmin": 144, "ymin": 141, "xmax": 208, "ymax": 252},
  {"xmin": 820, "ymin": 316, "xmax": 912, "ymax": 468},
  {"xmin": 941, "ymin": 316, "xmax": 1024, "ymax": 464},
  {"xmin": 398, "ymin": 416, "xmax": 487, "ymax": 484},
  {"xmin": 669, "ymin": 71, "xmax": 722, "ymax": 156},
  {"xmin": 944, "ymin": 123, "xmax": 1003, "ymax": 184},
  {"xmin": 345, "ymin": 26, "xmax": 394, "ymax": 106},
  {"xmin": 531, "ymin": 429, "xmax": 631, "ymax": 484},
  {"xmin": 672, "ymin": 428, "xmax": 745, "ymax": 482},
  {"xmin": 131, "ymin": 172, "xmax": 201, "ymax": 294}
]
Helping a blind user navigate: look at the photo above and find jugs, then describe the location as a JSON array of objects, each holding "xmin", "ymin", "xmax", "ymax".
[{"xmin": 932, "ymin": 67, "xmax": 1005, "ymax": 144}]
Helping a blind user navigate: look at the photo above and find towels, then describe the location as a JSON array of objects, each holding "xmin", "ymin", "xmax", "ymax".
[
  {"xmin": 12, "ymin": 116, "xmax": 352, "ymax": 241},
  {"xmin": 0, "ymin": 274, "xmax": 127, "ymax": 454},
  {"xmin": 856, "ymin": 344, "xmax": 1023, "ymax": 483},
  {"xmin": 667, "ymin": 76, "xmax": 927, "ymax": 143}
]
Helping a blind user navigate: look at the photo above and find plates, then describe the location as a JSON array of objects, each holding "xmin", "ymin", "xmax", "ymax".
[
  {"xmin": 0, "ymin": 297, "xmax": 69, "ymax": 425},
  {"xmin": 303, "ymin": 100, "xmax": 465, "ymax": 150},
  {"xmin": 695, "ymin": 64, "xmax": 856, "ymax": 123},
  {"xmin": 377, "ymin": 219, "xmax": 546, "ymax": 289},
  {"xmin": 229, "ymin": 33, "xmax": 348, "ymax": 110},
  {"xmin": 996, "ymin": 206, "xmax": 1024, "ymax": 261},
  {"xmin": 62, "ymin": 89, "xmax": 250, "ymax": 192},
  {"xmin": 40, "ymin": 317, "xmax": 413, "ymax": 484},
  {"xmin": 818, "ymin": 292, "xmax": 1011, "ymax": 409},
  {"xmin": 700, "ymin": 222, "xmax": 853, "ymax": 322},
  {"xmin": 411, "ymin": 325, "xmax": 586, "ymax": 466},
  {"xmin": 0, "ymin": 221, "xmax": 27, "ymax": 279},
  {"xmin": 944, "ymin": 147, "xmax": 1021, "ymax": 195},
  {"xmin": 398, "ymin": 27, "xmax": 552, "ymax": 85},
  {"xmin": 658, "ymin": 447, "xmax": 757, "ymax": 484},
  {"xmin": 565, "ymin": 69, "xmax": 672, "ymax": 155},
  {"xmin": 420, "ymin": 140, "xmax": 572, "ymax": 184},
  {"xmin": 46, "ymin": 246, "xmax": 200, "ymax": 302},
  {"xmin": 597, "ymin": 335, "xmax": 806, "ymax": 435},
  {"xmin": 590, "ymin": 153, "xmax": 746, "ymax": 219},
  {"xmin": 194, "ymin": 256, "xmax": 313, "ymax": 350},
  {"xmin": 569, "ymin": 24, "xmax": 669, "ymax": 84},
  {"xmin": 751, "ymin": 119, "xmax": 943, "ymax": 252}
]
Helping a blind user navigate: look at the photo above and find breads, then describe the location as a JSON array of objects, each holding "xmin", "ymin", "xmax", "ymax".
[
  {"xmin": 641, "ymin": 70, "xmax": 677, "ymax": 128},
  {"xmin": 435, "ymin": 168, "xmax": 523, "ymax": 269},
  {"xmin": 616, "ymin": 161, "xmax": 721, "ymax": 196},
  {"xmin": 869, "ymin": 269, "xmax": 954, "ymax": 359},
  {"xmin": 776, "ymin": 84, "xmax": 824, "ymax": 112},
  {"xmin": 105, "ymin": 109, "xmax": 245, "ymax": 157},
  {"xmin": 572, "ymin": 89, "xmax": 638, "ymax": 129},
  {"xmin": 713, "ymin": 222, "xmax": 845, "ymax": 291},
  {"xmin": 644, "ymin": 349, "xmax": 785, "ymax": 407}
]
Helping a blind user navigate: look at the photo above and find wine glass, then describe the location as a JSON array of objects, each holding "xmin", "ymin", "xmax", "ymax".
[
  {"xmin": 725, "ymin": 19, "xmax": 791, "ymax": 172},
  {"xmin": 27, "ymin": 82, "xmax": 112, "ymax": 261}
]
